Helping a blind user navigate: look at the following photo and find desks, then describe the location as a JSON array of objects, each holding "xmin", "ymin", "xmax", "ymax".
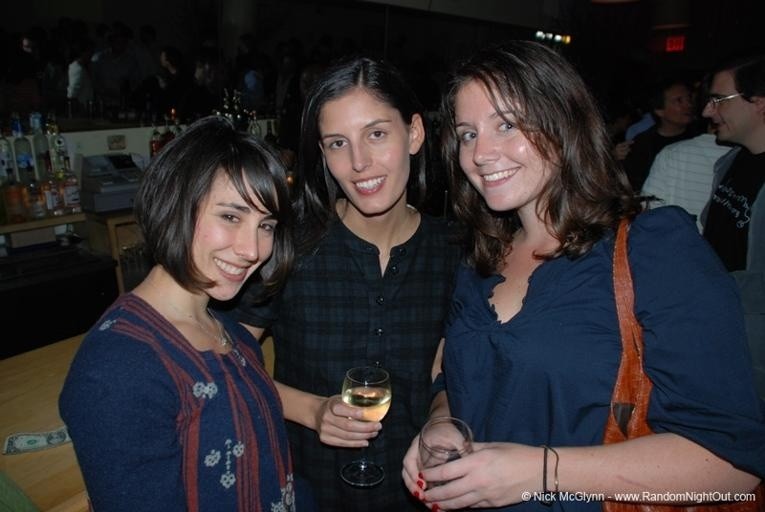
[
  {"xmin": 1, "ymin": 212, "xmax": 85, "ymax": 241},
  {"xmin": 0, "ymin": 330, "xmax": 275, "ymax": 512}
]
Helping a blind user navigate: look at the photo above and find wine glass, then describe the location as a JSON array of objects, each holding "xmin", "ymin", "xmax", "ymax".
[{"xmin": 337, "ymin": 364, "xmax": 393, "ymax": 489}]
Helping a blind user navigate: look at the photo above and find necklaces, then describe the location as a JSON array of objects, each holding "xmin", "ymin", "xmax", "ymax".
[{"xmin": 142, "ymin": 280, "xmax": 227, "ymax": 346}]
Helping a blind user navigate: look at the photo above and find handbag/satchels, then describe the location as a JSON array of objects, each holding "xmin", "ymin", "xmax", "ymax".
[{"xmin": 602, "ymin": 211, "xmax": 764, "ymax": 512}]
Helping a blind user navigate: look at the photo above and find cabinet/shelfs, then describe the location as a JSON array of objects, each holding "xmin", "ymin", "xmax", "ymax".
[{"xmin": 88, "ymin": 213, "xmax": 141, "ymax": 298}]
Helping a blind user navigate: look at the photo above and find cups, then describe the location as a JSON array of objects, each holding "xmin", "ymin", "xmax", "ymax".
[{"xmin": 416, "ymin": 416, "xmax": 475, "ymax": 493}]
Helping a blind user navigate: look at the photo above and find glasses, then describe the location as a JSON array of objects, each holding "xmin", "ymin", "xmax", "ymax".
[{"xmin": 705, "ymin": 93, "xmax": 745, "ymax": 109}]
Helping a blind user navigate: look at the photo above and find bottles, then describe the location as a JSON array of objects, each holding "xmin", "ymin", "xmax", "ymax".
[
  {"xmin": 149, "ymin": 88, "xmax": 276, "ymax": 163},
  {"xmin": 0, "ymin": 109, "xmax": 83, "ymax": 227}
]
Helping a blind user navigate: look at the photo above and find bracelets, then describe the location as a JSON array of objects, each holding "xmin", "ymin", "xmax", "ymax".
[{"xmin": 539, "ymin": 445, "xmax": 560, "ymax": 500}]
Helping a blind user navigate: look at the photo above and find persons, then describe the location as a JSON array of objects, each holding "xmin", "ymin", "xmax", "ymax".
[
  {"xmin": 59, "ymin": 114, "xmax": 318, "ymax": 512},
  {"xmin": 66, "ymin": 46, "xmax": 95, "ymax": 109},
  {"xmin": 607, "ymin": 47, "xmax": 765, "ymax": 406},
  {"xmin": 220, "ymin": 51, "xmax": 456, "ymax": 512},
  {"xmin": 155, "ymin": 47, "xmax": 182, "ymax": 91},
  {"xmin": 20, "ymin": 26, "xmax": 49, "ymax": 67},
  {"xmin": 401, "ymin": 34, "xmax": 765, "ymax": 512}
]
